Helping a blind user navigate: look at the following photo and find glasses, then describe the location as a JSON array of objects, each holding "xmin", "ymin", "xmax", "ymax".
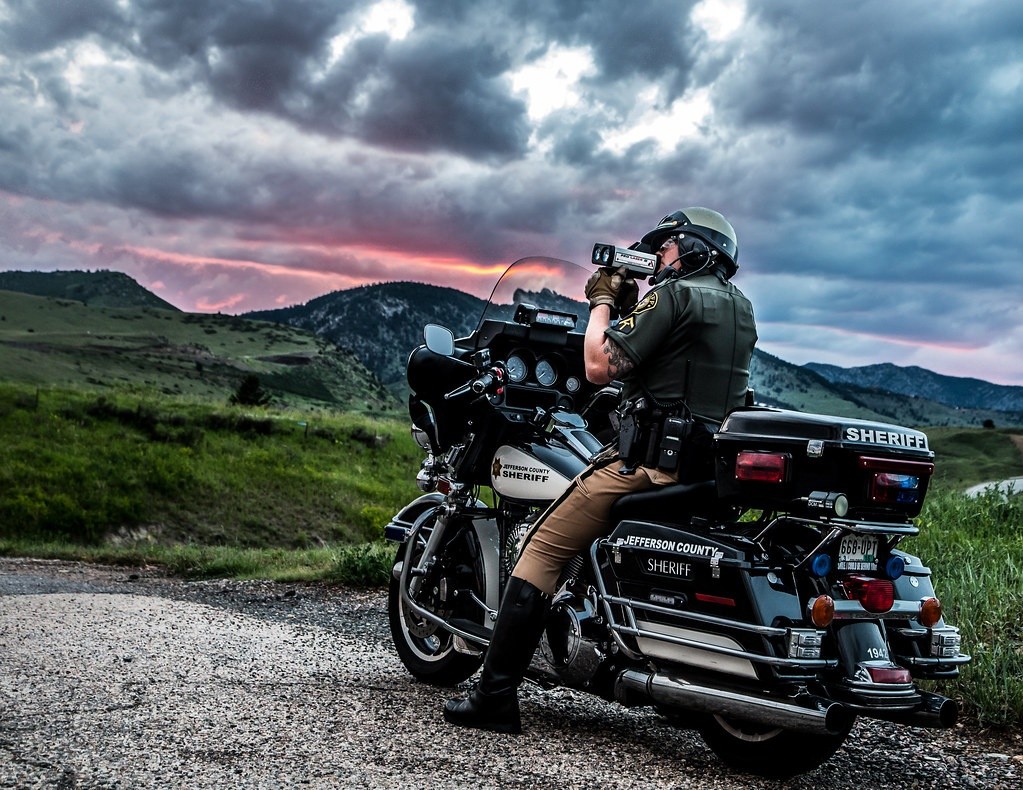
[{"xmin": 659, "ymin": 236, "xmax": 677, "ymax": 255}]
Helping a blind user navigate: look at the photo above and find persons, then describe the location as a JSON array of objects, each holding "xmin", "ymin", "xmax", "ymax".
[{"xmin": 442, "ymin": 208, "xmax": 758, "ymax": 737}]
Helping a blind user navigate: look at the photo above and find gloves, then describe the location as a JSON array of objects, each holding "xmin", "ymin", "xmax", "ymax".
[
  {"xmin": 615, "ymin": 277, "xmax": 638, "ymax": 318},
  {"xmin": 585, "ymin": 264, "xmax": 628, "ymax": 318}
]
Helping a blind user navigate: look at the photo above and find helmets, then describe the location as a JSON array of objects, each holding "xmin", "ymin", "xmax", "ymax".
[{"xmin": 641, "ymin": 207, "xmax": 739, "ymax": 279}]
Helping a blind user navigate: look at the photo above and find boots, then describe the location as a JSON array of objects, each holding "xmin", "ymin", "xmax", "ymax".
[{"xmin": 444, "ymin": 576, "xmax": 553, "ymax": 734}]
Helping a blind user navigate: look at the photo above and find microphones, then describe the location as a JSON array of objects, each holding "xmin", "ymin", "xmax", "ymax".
[{"xmin": 648, "ymin": 249, "xmax": 698, "ymax": 286}]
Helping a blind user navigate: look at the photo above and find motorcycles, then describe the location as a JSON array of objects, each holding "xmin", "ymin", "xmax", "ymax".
[{"xmin": 382, "ymin": 257, "xmax": 974, "ymax": 779}]
